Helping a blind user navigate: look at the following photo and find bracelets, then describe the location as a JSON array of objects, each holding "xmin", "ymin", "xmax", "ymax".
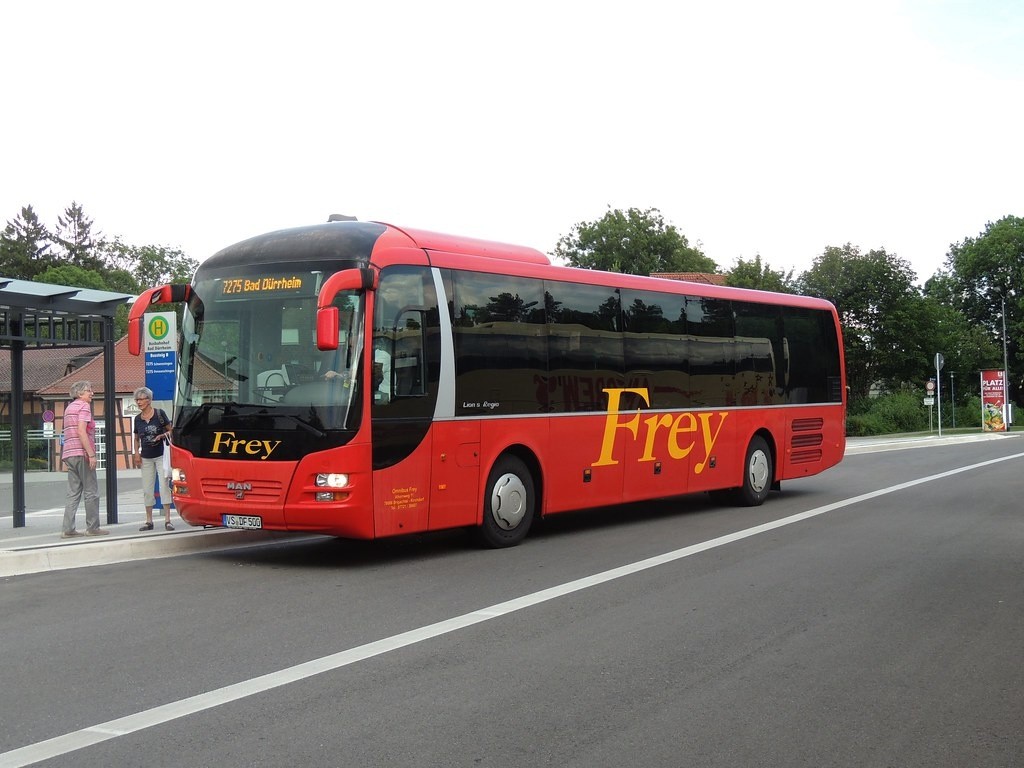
[{"xmin": 89, "ymin": 454, "xmax": 96, "ymax": 458}]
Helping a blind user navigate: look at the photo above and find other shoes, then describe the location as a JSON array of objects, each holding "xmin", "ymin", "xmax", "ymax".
[
  {"xmin": 61, "ymin": 529, "xmax": 84, "ymax": 538},
  {"xmin": 165, "ymin": 522, "xmax": 175, "ymax": 530},
  {"xmin": 139, "ymin": 522, "xmax": 153, "ymax": 531},
  {"xmin": 86, "ymin": 529, "xmax": 110, "ymax": 535}
]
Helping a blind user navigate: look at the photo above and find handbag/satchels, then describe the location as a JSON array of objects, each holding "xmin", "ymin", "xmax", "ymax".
[{"xmin": 162, "ymin": 432, "xmax": 172, "ymax": 479}]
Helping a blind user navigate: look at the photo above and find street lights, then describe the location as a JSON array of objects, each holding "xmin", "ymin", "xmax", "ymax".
[
  {"xmin": 945, "ymin": 371, "xmax": 958, "ymax": 428},
  {"xmin": 929, "ymin": 378, "xmax": 937, "ymax": 434},
  {"xmin": 1002, "ymin": 289, "xmax": 1018, "ymax": 431}
]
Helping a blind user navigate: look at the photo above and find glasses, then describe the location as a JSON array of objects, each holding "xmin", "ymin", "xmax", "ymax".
[{"xmin": 134, "ymin": 398, "xmax": 148, "ymax": 401}]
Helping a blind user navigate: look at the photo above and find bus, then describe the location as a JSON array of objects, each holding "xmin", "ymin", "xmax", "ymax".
[
  {"xmin": 128, "ymin": 215, "xmax": 847, "ymax": 548},
  {"xmin": 369, "ymin": 321, "xmax": 774, "ymax": 407}
]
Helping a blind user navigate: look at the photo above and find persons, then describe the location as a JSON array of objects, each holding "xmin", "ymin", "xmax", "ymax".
[
  {"xmin": 133, "ymin": 387, "xmax": 175, "ymax": 531},
  {"xmin": 62, "ymin": 380, "xmax": 110, "ymax": 538},
  {"xmin": 325, "ymin": 330, "xmax": 397, "ymax": 402}
]
